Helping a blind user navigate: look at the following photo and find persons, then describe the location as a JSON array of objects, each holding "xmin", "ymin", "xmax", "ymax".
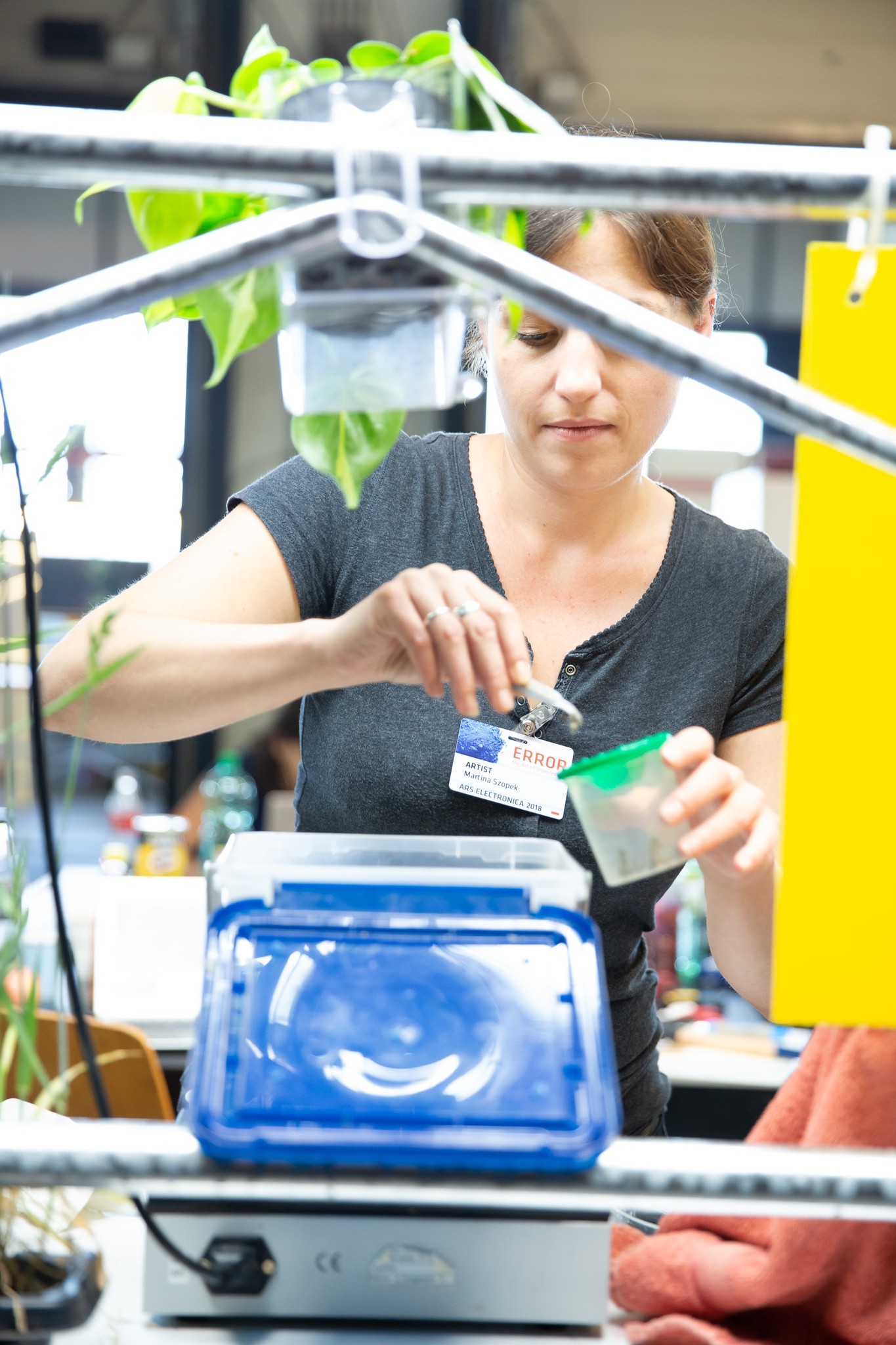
[
  {"xmin": 172, "ymin": 695, "xmax": 307, "ymax": 876},
  {"xmin": 37, "ymin": 123, "xmax": 824, "ymax": 1237}
]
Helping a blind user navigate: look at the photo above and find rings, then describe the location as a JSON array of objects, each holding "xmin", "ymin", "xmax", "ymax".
[
  {"xmin": 425, "ymin": 607, "xmax": 448, "ymax": 625},
  {"xmin": 454, "ymin": 602, "xmax": 481, "ymax": 616}
]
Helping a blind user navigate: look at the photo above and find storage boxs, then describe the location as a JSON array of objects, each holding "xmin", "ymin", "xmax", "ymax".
[{"xmin": 212, "ymin": 828, "xmax": 592, "ymax": 918}]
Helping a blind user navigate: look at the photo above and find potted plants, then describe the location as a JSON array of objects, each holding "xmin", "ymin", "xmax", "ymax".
[{"xmin": 73, "ymin": 19, "xmax": 593, "ymax": 512}]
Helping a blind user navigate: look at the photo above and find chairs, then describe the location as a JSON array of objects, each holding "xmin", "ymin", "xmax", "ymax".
[{"xmin": 0, "ymin": 1003, "xmax": 175, "ymax": 1122}]
